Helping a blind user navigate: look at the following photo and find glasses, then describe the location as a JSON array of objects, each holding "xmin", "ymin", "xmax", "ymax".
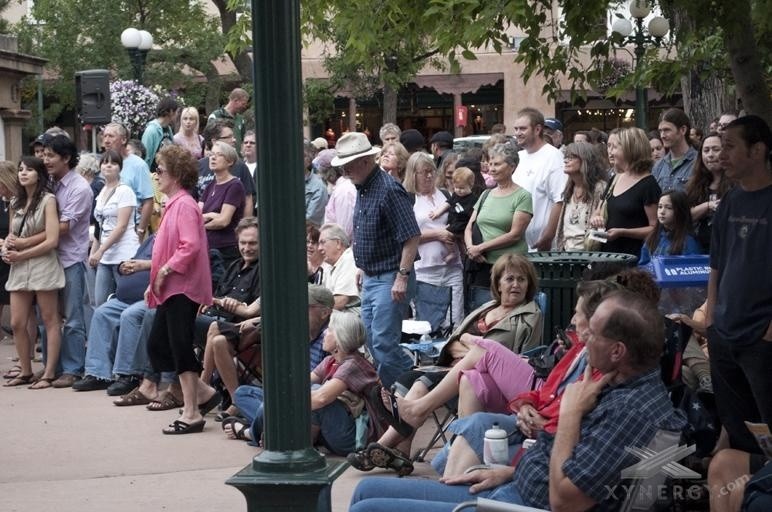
[
  {"xmin": 564, "ymin": 153, "xmax": 576, "ymax": 161},
  {"xmin": 218, "ymin": 134, "xmax": 233, "ymax": 140},
  {"xmin": 208, "ymin": 152, "xmax": 225, "ymax": 157},
  {"xmin": 156, "ymin": 168, "xmax": 168, "ymax": 175},
  {"xmin": 416, "ymin": 169, "xmax": 438, "ymax": 178},
  {"xmin": 318, "ymin": 238, "xmax": 340, "ymax": 246}
]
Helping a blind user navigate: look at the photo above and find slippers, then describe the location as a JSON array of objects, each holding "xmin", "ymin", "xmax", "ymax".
[
  {"xmin": 370, "ymin": 385, "xmax": 413, "ymax": 439},
  {"xmin": 2, "ymin": 365, "xmax": 56, "ymax": 390}
]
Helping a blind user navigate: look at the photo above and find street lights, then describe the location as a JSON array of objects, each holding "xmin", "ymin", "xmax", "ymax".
[
  {"xmin": 118, "ymin": 22, "xmax": 156, "ymax": 84},
  {"xmin": 608, "ymin": 0, "xmax": 674, "ymax": 124}
]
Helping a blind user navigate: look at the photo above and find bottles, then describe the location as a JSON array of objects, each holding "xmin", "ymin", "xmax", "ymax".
[
  {"xmin": 417, "ymin": 332, "xmax": 434, "ymax": 367},
  {"xmin": 482, "ymin": 423, "xmax": 510, "ymax": 466}
]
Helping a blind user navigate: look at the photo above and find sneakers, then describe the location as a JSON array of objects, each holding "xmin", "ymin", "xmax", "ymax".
[
  {"xmin": 51, "ymin": 373, "xmax": 140, "ymax": 396},
  {"xmin": 346, "ymin": 443, "xmax": 414, "ymax": 476}
]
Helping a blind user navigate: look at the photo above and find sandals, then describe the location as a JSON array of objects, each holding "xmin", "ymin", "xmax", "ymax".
[{"xmin": 113, "ymin": 385, "xmax": 251, "ymax": 440}]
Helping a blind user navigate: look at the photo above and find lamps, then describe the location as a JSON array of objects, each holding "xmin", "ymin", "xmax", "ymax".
[{"xmin": 625, "ymin": 109, "xmax": 634, "ymax": 118}]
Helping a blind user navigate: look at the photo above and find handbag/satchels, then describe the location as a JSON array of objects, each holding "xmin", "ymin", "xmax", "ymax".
[
  {"xmin": 585, "ymin": 199, "xmax": 609, "ymax": 252},
  {"xmin": 464, "ymin": 221, "xmax": 486, "ymax": 275}
]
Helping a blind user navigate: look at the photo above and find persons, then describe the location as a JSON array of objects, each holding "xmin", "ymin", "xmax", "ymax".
[
  {"xmin": 298, "ymin": 106, "xmax": 771, "ymax": 511},
  {"xmin": 0, "ymin": 86, "xmax": 265, "ymax": 449}
]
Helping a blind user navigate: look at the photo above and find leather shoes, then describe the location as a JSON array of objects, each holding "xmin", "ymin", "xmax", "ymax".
[{"xmin": 1, "ymin": 325, "xmax": 13, "ymax": 336}]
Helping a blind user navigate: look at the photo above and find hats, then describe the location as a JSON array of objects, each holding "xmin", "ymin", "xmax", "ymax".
[
  {"xmin": 399, "ymin": 129, "xmax": 425, "ymax": 152},
  {"xmin": 428, "ymin": 132, "xmax": 453, "ymax": 144},
  {"xmin": 310, "ymin": 137, "xmax": 328, "ymax": 150},
  {"xmin": 331, "ymin": 132, "xmax": 380, "ymax": 167},
  {"xmin": 311, "ymin": 148, "xmax": 336, "ymax": 168},
  {"xmin": 29, "ymin": 133, "xmax": 53, "ymax": 146},
  {"xmin": 544, "ymin": 118, "xmax": 562, "ymax": 131}
]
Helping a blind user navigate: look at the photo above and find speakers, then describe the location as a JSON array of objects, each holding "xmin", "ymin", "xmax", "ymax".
[{"xmin": 75, "ymin": 69, "xmax": 111, "ymax": 125}]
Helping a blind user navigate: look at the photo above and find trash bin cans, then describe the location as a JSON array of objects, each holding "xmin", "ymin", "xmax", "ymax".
[{"xmin": 523, "ymin": 251, "xmax": 638, "ymax": 346}]
